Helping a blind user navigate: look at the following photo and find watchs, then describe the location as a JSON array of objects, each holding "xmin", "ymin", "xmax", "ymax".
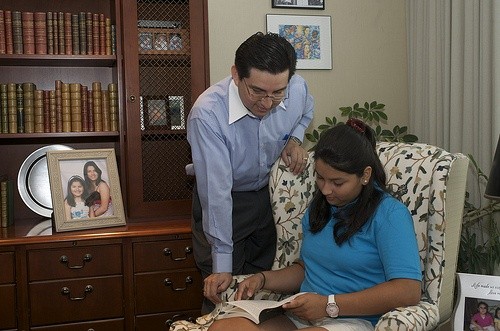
[
  {"xmin": 290, "ymin": 136, "xmax": 301, "ymax": 145},
  {"xmin": 326, "ymin": 294, "xmax": 339, "ymax": 318}
]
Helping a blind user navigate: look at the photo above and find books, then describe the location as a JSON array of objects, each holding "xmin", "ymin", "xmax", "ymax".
[
  {"xmin": 0, "ymin": 10, "xmax": 47, "ymax": 54},
  {"xmin": 92, "ymin": 81, "xmax": 118, "ymax": 131},
  {"xmin": 0, "ymin": 177, "xmax": 14, "ymax": 228},
  {"xmin": 55, "ymin": 79, "xmax": 93, "ymax": 133},
  {"xmin": 0, "ymin": 83, "xmax": 18, "ymax": 133},
  {"xmin": 216, "ymin": 290, "xmax": 316, "ymax": 325},
  {"xmin": 16, "ymin": 82, "xmax": 35, "ymax": 133},
  {"xmin": 79, "ymin": 11, "xmax": 115, "ymax": 55},
  {"xmin": 46, "ymin": 12, "xmax": 80, "ymax": 55},
  {"xmin": 33, "ymin": 90, "xmax": 57, "ymax": 133}
]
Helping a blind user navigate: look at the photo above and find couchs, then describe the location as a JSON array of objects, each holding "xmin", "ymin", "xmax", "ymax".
[{"xmin": 213, "ymin": 142, "xmax": 470, "ymax": 331}]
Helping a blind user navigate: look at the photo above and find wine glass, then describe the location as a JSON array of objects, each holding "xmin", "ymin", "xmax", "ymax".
[{"xmin": 137, "ymin": 20, "xmax": 182, "ymax": 51}]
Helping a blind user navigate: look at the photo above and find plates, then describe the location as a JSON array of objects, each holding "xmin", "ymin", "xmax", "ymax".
[{"xmin": 16, "ymin": 145, "xmax": 75, "ymax": 218}]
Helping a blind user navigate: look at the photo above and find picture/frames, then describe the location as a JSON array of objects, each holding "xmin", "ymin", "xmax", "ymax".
[
  {"xmin": 46, "ymin": 147, "xmax": 127, "ymax": 232},
  {"xmin": 266, "ymin": 14, "xmax": 333, "ymax": 71},
  {"xmin": 453, "ymin": 273, "xmax": 499, "ymax": 330},
  {"xmin": 271, "ymin": 0, "xmax": 325, "ymax": 11},
  {"xmin": 140, "ymin": 96, "xmax": 185, "ymax": 130},
  {"xmin": 139, "ymin": 27, "xmax": 190, "ymax": 56},
  {"xmin": 144, "ymin": 93, "xmax": 171, "ymax": 130}
]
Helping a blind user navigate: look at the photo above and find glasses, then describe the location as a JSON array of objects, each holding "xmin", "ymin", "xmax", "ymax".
[{"xmin": 244, "ymin": 80, "xmax": 291, "ymax": 102}]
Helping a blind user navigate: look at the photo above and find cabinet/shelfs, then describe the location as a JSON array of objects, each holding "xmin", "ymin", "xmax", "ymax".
[
  {"xmin": 4, "ymin": 0, "xmax": 211, "ymax": 222},
  {"xmin": 0, "ymin": 201, "xmax": 203, "ymax": 331}
]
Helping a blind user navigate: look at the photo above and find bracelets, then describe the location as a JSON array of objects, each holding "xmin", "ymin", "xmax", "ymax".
[{"xmin": 256, "ymin": 272, "xmax": 265, "ymax": 290}]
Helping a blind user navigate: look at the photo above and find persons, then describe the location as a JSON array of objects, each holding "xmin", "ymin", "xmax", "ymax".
[
  {"xmin": 82, "ymin": 161, "xmax": 114, "ymax": 217},
  {"xmin": 471, "ymin": 302, "xmax": 495, "ymax": 331},
  {"xmin": 208, "ymin": 119, "xmax": 422, "ymax": 331},
  {"xmin": 187, "ymin": 34, "xmax": 313, "ymax": 314},
  {"xmin": 64, "ymin": 175, "xmax": 95, "ymax": 219},
  {"xmin": 493, "ymin": 308, "xmax": 500, "ymax": 331}
]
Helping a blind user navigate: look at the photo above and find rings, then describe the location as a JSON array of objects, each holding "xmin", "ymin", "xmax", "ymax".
[{"xmin": 303, "ymin": 157, "xmax": 308, "ymax": 161}]
